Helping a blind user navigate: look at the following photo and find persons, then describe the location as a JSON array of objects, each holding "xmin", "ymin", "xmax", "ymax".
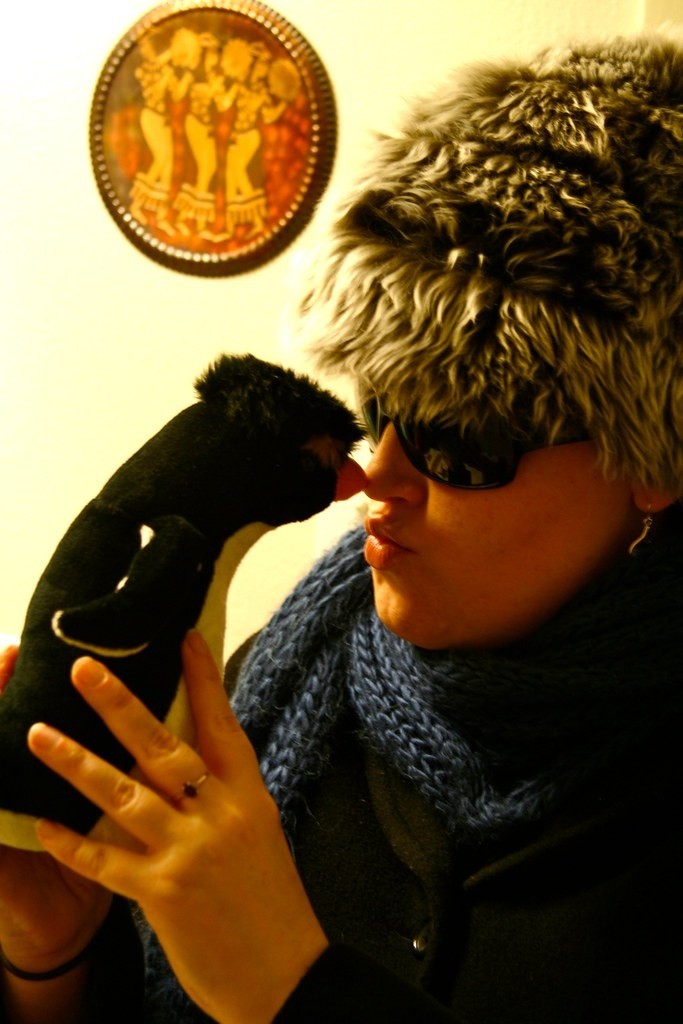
[{"xmin": 0, "ymin": 33, "xmax": 683, "ymax": 1024}]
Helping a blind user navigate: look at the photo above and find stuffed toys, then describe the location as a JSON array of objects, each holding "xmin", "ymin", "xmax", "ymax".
[{"xmin": 0, "ymin": 353, "xmax": 373, "ymax": 857}]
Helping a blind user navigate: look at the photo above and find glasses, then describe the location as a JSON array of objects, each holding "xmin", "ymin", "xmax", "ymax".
[{"xmin": 365, "ymin": 393, "xmax": 591, "ymax": 490}]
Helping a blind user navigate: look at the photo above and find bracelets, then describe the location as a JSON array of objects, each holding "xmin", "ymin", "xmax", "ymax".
[{"xmin": 1, "ymin": 921, "xmax": 113, "ymax": 983}]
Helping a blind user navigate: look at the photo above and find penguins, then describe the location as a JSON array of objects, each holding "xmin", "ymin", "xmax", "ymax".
[{"xmin": 0, "ymin": 352, "xmax": 374, "ymax": 855}]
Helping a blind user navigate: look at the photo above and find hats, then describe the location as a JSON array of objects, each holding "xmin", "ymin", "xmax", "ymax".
[{"xmin": 298, "ymin": 34, "xmax": 683, "ymax": 491}]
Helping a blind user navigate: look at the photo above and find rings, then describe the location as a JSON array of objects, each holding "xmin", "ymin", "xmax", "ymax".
[{"xmin": 170, "ymin": 771, "xmax": 210, "ymax": 803}]
